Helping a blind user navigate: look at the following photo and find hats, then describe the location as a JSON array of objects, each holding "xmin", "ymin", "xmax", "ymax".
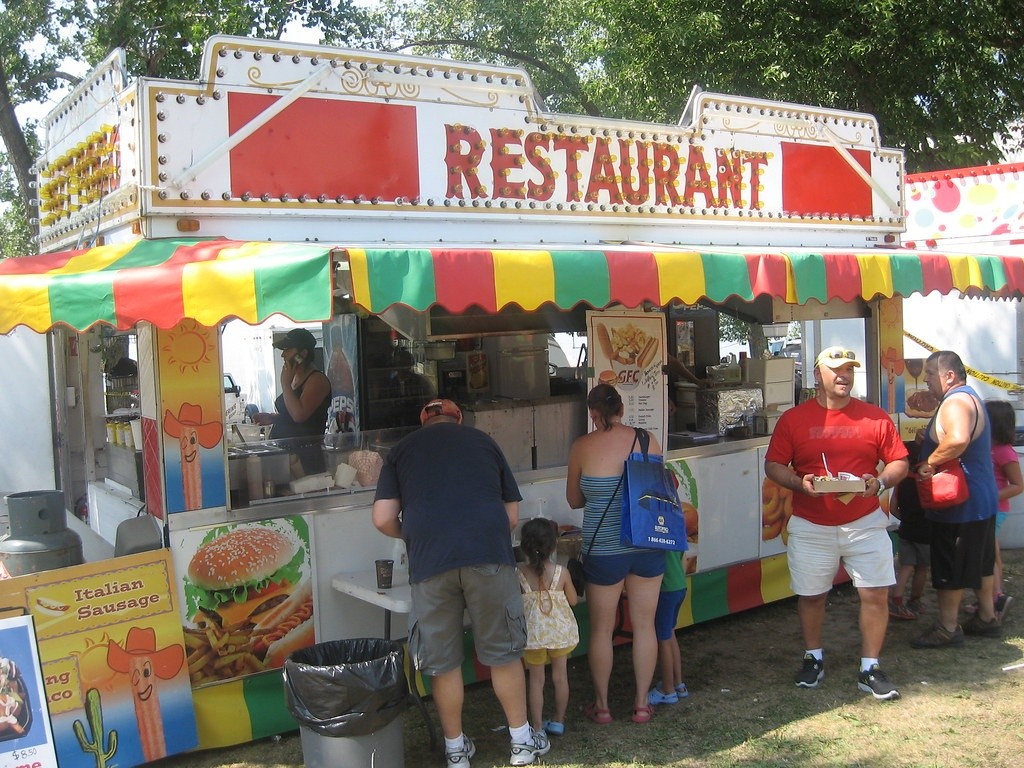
[
  {"xmin": 273, "ymin": 329, "xmax": 316, "ymax": 350},
  {"xmin": 814, "ymin": 346, "xmax": 861, "ymax": 368}
]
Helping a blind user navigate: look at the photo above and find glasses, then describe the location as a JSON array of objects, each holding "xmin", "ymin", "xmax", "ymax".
[{"xmin": 814, "ymin": 350, "xmax": 855, "ymax": 366}]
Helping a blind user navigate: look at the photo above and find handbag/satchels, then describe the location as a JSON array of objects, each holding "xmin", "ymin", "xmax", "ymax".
[
  {"xmin": 913, "ymin": 457, "xmax": 970, "ymax": 511},
  {"xmin": 620, "ymin": 427, "xmax": 689, "ymax": 552},
  {"xmin": 567, "ymin": 558, "xmax": 585, "ymax": 597}
]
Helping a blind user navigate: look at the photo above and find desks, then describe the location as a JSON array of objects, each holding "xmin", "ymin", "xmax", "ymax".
[{"xmin": 332, "ymin": 564, "xmax": 634, "ymax": 752}]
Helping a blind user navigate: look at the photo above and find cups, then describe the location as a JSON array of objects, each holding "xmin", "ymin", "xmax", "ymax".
[
  {"xmin": 374, "ymin": 559, "xmax": 394, "ymax": 589},
  {"xmin": 105, "ymin": 415, "xmax": 143, "ymax": 451}
]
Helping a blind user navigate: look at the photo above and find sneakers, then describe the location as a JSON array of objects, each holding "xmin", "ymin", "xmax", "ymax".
[
  {"xmin": 993, "ymin": 591, "xmax": 1013, "ymax": 620},
  {"xmin": 888, "ymin": 602, "xmax": 917, "ymax": 620},
  {"xmin": 906, "ymin": 599, "xmax": 933, "ymax": 614},
  {"xmin": 857, "ymin": 663, "xmax": 901, "ymax": 700},
  {"xmin": 910, "ymin": 619, "xmax": 964, "ymax": 649},
  {"xmin": 965, "ymin": 601, "xmax": 978, "ymax": 614},
  {"xmin": 446, "ymin": 734, "xmax": 476, "ymax": 768},
  {"xmin": 509, "ymin": 727, "xmax": 551, "ymax": 766},
  {"xmin": 961, "ymin": 616, "xmax": 1002, "ymax": 638},
  {"xmin": 794, "ymin": 652, "xmax": 825, "ymax": 687}
]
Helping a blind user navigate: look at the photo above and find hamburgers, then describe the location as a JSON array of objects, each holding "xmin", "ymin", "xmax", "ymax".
[
  {"xmin": 184, "ymin": 515, "xmax": 314, "ymax": 669},
  {"xmin": 599, "ymin": 370, "xmax": 617, "ymax": 386}
]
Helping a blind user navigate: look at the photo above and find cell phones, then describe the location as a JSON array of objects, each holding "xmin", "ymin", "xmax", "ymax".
[{"xmin": 289, "ymin": 353, "xmax": 305, "ymax": 368}]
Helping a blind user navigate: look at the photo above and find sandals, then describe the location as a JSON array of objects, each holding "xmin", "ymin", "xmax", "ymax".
[
  {"xmin": 657, "ymin": 681, "xmax": 689, "ymax": 698},
  {"xmin": 647, "ymin": 688, "xmax": 678, "ymax": 705},
  {"xmin": 542, "ymin": 720, "xmax": 564, "ymax": 735},
  {"xmin": 582, "ymin": 703, "xmax": 612, "ymax": 724},
  {"xmin": 630, "ymin": 703, "xmax": 655, "ymax": 721}
]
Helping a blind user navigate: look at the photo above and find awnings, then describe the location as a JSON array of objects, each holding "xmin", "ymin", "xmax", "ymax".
[{"xmin": 0, "ymin": 236, "xmax": 1024, "ymax": 337}]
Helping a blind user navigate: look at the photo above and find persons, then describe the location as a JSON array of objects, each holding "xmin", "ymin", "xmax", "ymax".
[
  {"xmin": 890, "ymin": 351, "xmax": 1024, "ymax": 648},
  {"xmin": 254, "ymin": 328, "xmax": 332, "ymax": 477},
  {"xmin": 515, "ymin": 517, "xmax": 579, "ymax": 735},
  {"xmin": 764, "ymin": 346, "xmax": 909, "ymax": 701},
  {"xmin": 372, "ymin": 398, "xmax": 551, "ymax": 768},
  {"xmin": 669, "ymin": 355, "xmax": 711, "ymax": 416},
  {"xmin": 566, "ymin": 385, "xmax": 666, "ymax": 722},
  {"xmin": 648, "ymin": 550, "xmax": 688, "ymax": 705}
]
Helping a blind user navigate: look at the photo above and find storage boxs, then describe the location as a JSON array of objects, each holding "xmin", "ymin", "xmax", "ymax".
[{"xmin": 696, "ymin": 358, "xmax": 796, "ymax": 435}]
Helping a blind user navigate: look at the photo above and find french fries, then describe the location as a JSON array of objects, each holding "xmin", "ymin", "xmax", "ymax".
[{"xmin": 184, "ymin": 619, "xmax": 270, "ymax": 683}]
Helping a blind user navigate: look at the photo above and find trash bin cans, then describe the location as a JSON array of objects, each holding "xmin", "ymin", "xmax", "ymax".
[{"xmin": 286, "ymin": 638, "xmax": 404, "ymax": 768}]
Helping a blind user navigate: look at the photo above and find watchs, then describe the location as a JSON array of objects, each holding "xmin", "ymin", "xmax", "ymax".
[{"xmin": 874, "ymin": 478, "xmax": 886, "ymax": 496}]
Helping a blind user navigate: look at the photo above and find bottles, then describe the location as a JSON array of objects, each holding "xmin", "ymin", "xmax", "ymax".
[{"xmin": 327, "ymin": 327, "xmax": 354, "ymax": 451}]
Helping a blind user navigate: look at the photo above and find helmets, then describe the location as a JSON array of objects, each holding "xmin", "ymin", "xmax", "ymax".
[{"xmin": 420, "ymin": 399, "xmax": 462, "ymax": 426}]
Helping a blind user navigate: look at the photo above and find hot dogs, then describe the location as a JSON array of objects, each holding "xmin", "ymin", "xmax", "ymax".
[{"xmin": 634, "ymin": 337, "xmax": 658, "ymax": 369}]
